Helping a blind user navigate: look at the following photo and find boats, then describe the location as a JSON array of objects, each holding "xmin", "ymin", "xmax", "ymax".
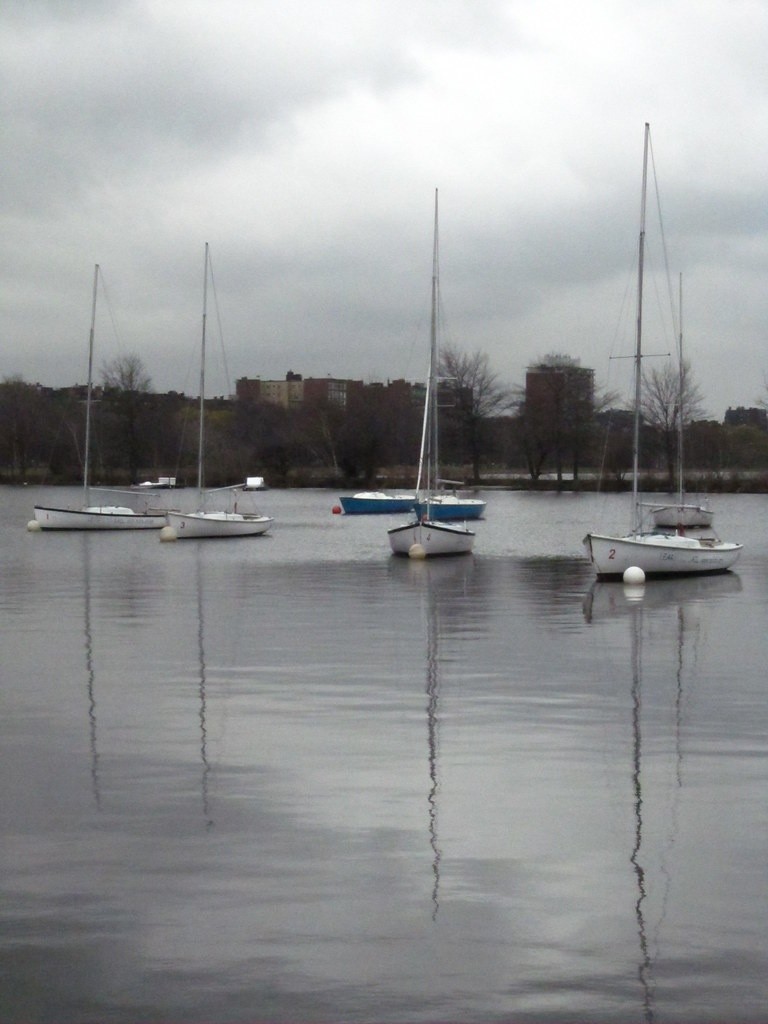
[
  {"xmin": 339, "ymin": 494, "xmax": 418, "ymax": 514},
  {"xmin": 413, "ymin": 368, "xmax": 486, "ymax": 521}
]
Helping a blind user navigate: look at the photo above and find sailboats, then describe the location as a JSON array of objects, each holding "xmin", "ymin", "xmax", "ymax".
[
  {"xmin": 388, "ymin": 188, "xmax": 476, "ymax": 557},
  {"xmin": 652, "ymin": 272, "xmax": 714, "ymax": 526},
  {"xmin": 33, "ymin": 264, "xmax": 168, "ymax": 530},
  {"xmin": 166, "ymin": 243, "xmax": 273, "ymax": 539},
  {"xmin": 582, "ymin": 123, "xmax": 742, "ymax": 582}
]
